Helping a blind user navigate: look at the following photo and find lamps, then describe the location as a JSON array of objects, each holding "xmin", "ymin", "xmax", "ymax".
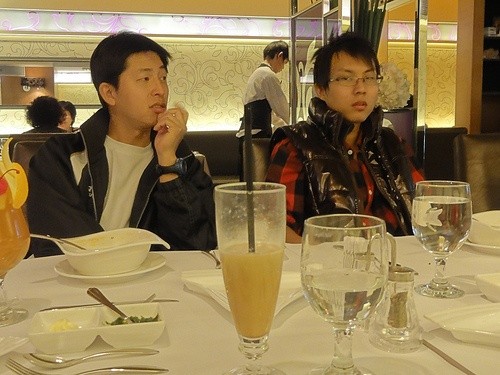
[{"xmin": 21, "ymin": 78, "xmax": 45, "ymax": 92}]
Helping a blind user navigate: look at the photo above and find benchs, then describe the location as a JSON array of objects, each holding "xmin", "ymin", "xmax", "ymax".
[{"xmin": 184, "ymin": 131, "xmax": 242, "ymax": 187}]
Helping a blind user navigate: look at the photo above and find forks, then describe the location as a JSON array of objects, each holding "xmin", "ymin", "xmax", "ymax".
[
  {"xmin": 5, "ymin": 358, "xmax": 169, "ymax": 375},
  {"xmin": 200, "ymin": 250, "xmax": 221, "ymax": 270}
]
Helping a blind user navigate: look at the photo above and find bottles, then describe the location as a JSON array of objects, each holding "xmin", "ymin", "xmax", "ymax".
[{"xmin": 365, "ymin": 261, "xmax": 425, "ymax": 352}]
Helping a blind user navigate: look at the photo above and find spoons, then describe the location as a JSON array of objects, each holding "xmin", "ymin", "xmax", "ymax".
[
  {"xmin": 86, "ymin": 288, "xmax": 135, "ymax": 324},
  {"xmin": 29, "ymin": 349, "xmax": 161, "ymax": 367}
]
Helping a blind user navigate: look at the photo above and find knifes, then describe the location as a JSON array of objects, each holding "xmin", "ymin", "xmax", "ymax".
[
  {"xmin": 39, "ymin": 299, "xmax": 180, "ymax": 312},
  {"xmin": 420, "ymin": 339, "xmax": 476, "ymax": 375}
]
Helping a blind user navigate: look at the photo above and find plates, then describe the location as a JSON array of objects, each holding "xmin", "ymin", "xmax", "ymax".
[
  {"xmin": 53, "ymin": 253, "xmax": 168, "ymax": 284},
  {"xmin": 463, "ymin": 239, "xmax": 500, "ymax": 254},
  {"xmin": 424, "ymin": 301, "xmax": 500, "ymax": 347}
]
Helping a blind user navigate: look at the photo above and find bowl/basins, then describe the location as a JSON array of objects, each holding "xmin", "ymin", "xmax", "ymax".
[
  {"xmin": 55, "ymin": 227, "xmax": 171, "ymax": 275},
  {"xmin": 465, "ymin": 210, "xmax": 500, "ymax": 246},
  {"xmin": 98, "ymin": 302, "xmax": 168, "ymax": 348},
  {"xmin": 26, "ymin": 304, "xmax": 98, "ymax": 352},
  {"xmin": 474, "ymin": 272, "xmax": 500, "ymax": 301}
]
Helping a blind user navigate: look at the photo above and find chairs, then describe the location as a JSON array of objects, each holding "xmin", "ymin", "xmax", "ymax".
[
  {"xmin": 243, "ymin": 138, "xmax": 271, "ymax": 190},
  {"xmin": 455, "ymin": 134, "xmax": 500, "ymax": 214},
  {"xmin": 427, "ymin": 127, "xmax": 468, "ymax": 181},
  {"xmin": 9, "ymin": 133, "xmax": 76, "ymax": 176}
]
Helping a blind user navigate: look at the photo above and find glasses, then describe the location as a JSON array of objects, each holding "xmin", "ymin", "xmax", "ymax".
[{"xmin": 330, "ymin": 73, "xmax": 383, "ymax": 86}]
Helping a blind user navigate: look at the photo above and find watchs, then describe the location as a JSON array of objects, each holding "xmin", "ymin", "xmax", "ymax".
[{"xmin": 155, "ymin": 157, "xmax": 188, "ymax": 178}]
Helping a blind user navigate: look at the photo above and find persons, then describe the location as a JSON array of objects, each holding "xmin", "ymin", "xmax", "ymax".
[
  {"xmin": 261, "ymin": 30, "xmax": 428, "ymax": 244},
  {"xmin": 22, "ymin": 96, "xmax": 81, "ymax": 134},
  {"xmin": 24, "ymin": 31, "xmax": 219, "ymax": 259},
  {"xmin": 234, "ymin": 40, "xmax": 290, "ymax": 182}
]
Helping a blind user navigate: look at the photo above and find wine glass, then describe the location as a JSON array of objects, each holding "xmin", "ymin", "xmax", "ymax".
[
  {"xmin": 412, "ymin": 180, "xmax": 473, "ymax": 298},
  {"xmin": 1, "ymin": 176, "xmax": 31, "ymax": 328},
  {"xmin": 214, "ymin": 182, "xmax": 287, "ymax": 375},
  {"xmin": 298, "ymin": 214, "xmax": 388, "ymax": 375}
]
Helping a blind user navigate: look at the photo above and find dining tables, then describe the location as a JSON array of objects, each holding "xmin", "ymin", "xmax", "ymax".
[{"xmin": 0, "ymin": 234, "xmax": 500, "ymax": 375}]
